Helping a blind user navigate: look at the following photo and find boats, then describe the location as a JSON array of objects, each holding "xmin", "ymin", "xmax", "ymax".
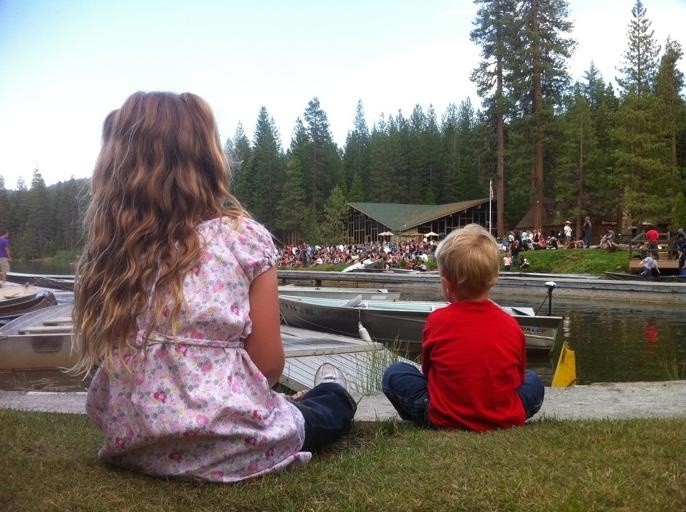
[
  {"xmin": 0, "ymin": 280, "xmax": 56, "ymax": 323},
  {"xmin": 276, "ymin": 281, "xmax": 575, "ymax": 363},
  {"xmin": 273, "ymin": 284, "xmax": 404, "ymax": 300},
  {"xmin": 0, "ymin": 297, "xmax": 92, "ymax": 374}
]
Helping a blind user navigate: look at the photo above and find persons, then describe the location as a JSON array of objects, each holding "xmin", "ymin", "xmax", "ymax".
[
  {"xmin": 69, "ymin": 91, "xmax": 358, "ymax": 483},
  {"xmin": 645, "ymin": 317, "xmax": 659, "ymax": 353},
  {"xmin": 676, "ymin": 228, "xmax": 686, "ymax": 275},
  {"xmin": 0, "ymin": 229, "xmax": 12, "ymax": 286},
  {"xmin": 497, "ymin": 216, "xmax": 661, "ymax": 277},
  {"xmin": 381, "ymin": 223, "xmax": 545, "ymax": 432},
  {"xmin": 276, "ymin": 236, "xmax": 437, "ymax": 271}
]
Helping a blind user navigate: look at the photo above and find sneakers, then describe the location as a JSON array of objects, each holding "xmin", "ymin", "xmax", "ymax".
[{"xmin": 314, "ymin": 363, "xmax": 347, "ymax": 392}]
